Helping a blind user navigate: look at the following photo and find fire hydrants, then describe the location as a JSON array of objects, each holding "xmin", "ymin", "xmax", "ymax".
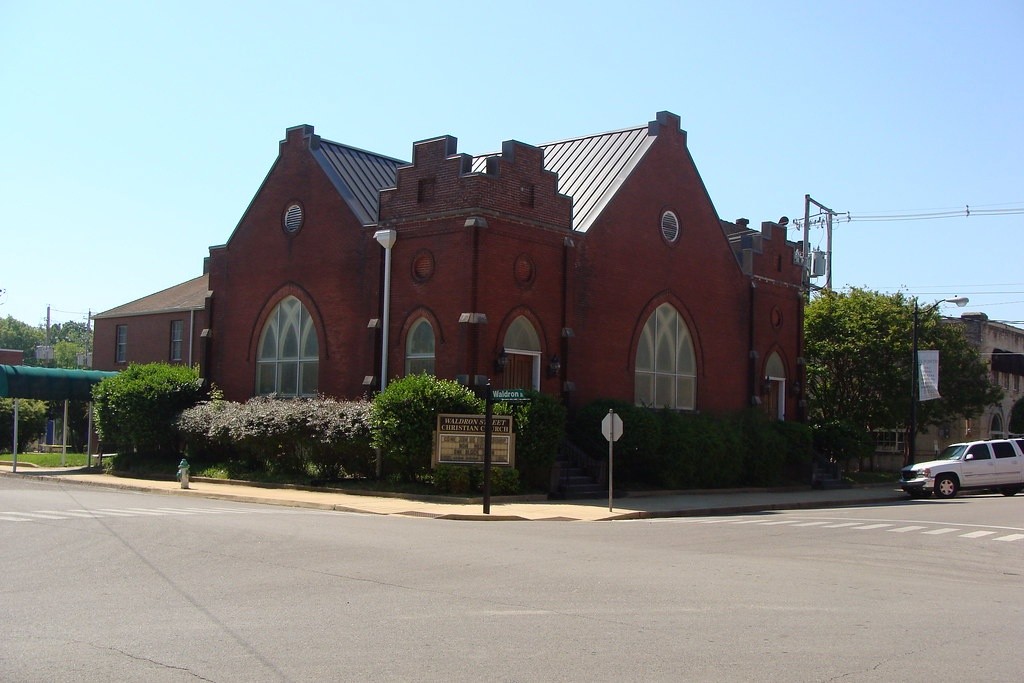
[{"xmin": 175, "ymin": 458, "xmax": 191, "ymax": 489}]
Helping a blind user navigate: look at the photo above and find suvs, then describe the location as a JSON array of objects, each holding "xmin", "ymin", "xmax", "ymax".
[{"xmin": 898, "ymin": 436, "xmax": 1024, "ymax": 499}]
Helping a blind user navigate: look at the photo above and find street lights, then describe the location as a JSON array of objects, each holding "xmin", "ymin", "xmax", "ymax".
[
  {"xmin": 906, "ymin": 296, "xmax": 970, "ymax": 466},
  {"xmin": 373, "ymin": 229, "xmax": 398, "ymax": 479}
]
